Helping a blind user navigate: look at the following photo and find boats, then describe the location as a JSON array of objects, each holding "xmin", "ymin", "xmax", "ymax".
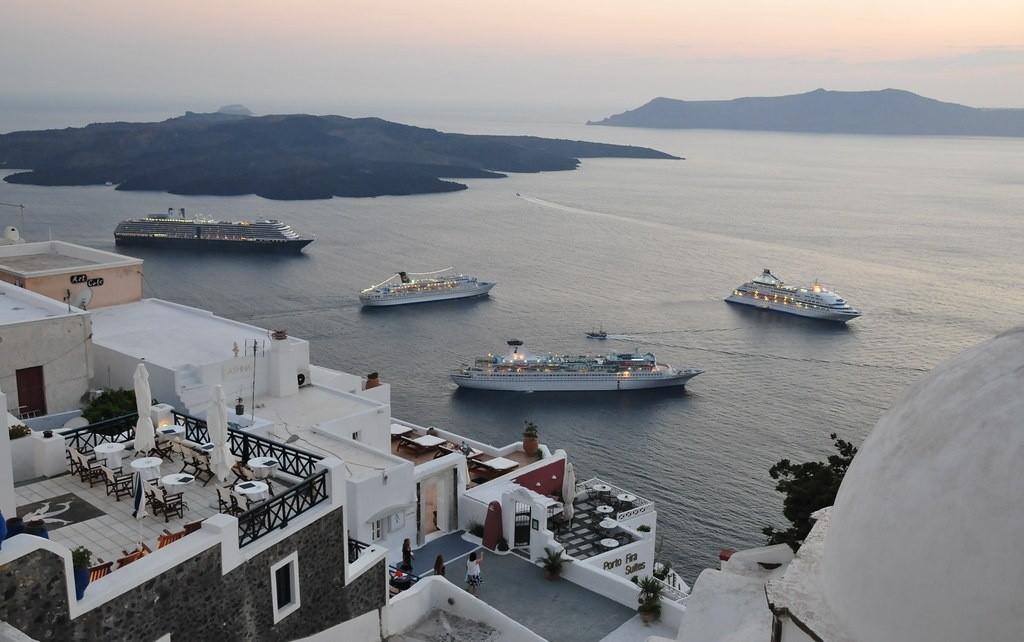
[
  {"xmin": 505, "ymin": 338, "xmax": 524, "ymax": 346},
  {"xmin": 113, "ymin": 206, "xmax": 316, "ymax": 253},
  {"xmin": 356, "ymin": 266, "xmax": 498, "ymax": 308},
  {"xmin": 586, "ymin": 323, "xmax": 607, "ymax": 339},
  {"xmin": 449, "ymin": 347, "xmax": 709, "ymax": 392},
  {"xmin": 722, "ymin": 269, "xmax": 863, "ymax": 323}
]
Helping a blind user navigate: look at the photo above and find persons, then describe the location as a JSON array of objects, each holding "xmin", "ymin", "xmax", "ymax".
[
  {"xmin": 401, "ymin": 538, "xmax": 415, "ymax": 574},
  {"xmin": 465, "ymin": 551, "xmax": 484, "ymax": 598},
  {"xmin": 433, "ymin": 555, "xmax": 446, "ymax": 577}
]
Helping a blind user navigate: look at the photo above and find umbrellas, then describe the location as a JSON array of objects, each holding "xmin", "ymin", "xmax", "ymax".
[
  {"xmin": 562, "ymin": 463, "xmax": 576, "ymax": 531},
  {"xmin": 131, "ymin": 471, "xmax": 148, "ymax": 552},
  {"xmin": 133, "ymin": 363, "xmax": 156, "ymax": 459},
  {"xmin": 205, "ymin": 384, "xmax": 236, "ymax": 483}
]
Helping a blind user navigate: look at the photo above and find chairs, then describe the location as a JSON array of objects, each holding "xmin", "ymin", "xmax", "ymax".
[
  {"xmin": 366, "ymin": 370, "xmax": 379, "ymax": 389},
  {"xmin": 584, "ymin": 484, "xmax": 632, "ymax": 556},
  {"xmin": 472, "ymin": 456, "xmax": 519, "ymax": 476},
  {"xmin": 434, "ymin": 445, "xmax": 485, "ymax": 471},
  {"xmin": 87, "ymin": 517, "xmax": 209, "ymax": 586},
  {"xmin": 391, "ymin": 424, "xmax": 413, "ymax": 441},
  {"xmin": 397, "ymin": 435, "xmax": 446, "ymax": 459},
  {"xmin": 66, "ymin": 440, "xmax": 280, "ymax": 524},
  {"xmin": 389, "ymin": 584, "xmax": 399, "ymax": 597}
]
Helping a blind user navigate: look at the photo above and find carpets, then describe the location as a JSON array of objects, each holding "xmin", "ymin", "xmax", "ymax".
[{"xmin": 16, "ymin": 491, "xmax": 107, "ymax": 532}]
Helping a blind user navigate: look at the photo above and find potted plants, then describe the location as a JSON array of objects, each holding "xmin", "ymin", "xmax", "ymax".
[
  {"xmin": 522, "ymin": 421, "xmax": 538, "ymax": 457},
  {"xmin": 535, "ymin": 547, "xmax": 574, "ymax": 580},
  {"xmin": 635, "ymin": 574, "xmax": 665, "ymax": 622},
  {"xmin": 235, "ymin": 397, "xmax": 245, "ymax": 415},
  {"xmin": 69, "ymin": 545, "xmax": 92, "ymax": 600}
]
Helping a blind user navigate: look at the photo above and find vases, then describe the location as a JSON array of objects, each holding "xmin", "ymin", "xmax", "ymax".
[{"xmin": 43, "ymin": 431, "xmax": 54, "ymax": 438}]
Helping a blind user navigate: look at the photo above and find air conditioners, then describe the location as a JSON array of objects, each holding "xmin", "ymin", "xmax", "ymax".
[{"xmin": 297, "ymin": 368, "xmax": 313, "ymax": 389}]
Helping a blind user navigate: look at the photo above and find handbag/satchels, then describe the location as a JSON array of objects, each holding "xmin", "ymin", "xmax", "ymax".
[{"xmin": 400, "ymin": 565, "xmax": 407, "ymax": 571}]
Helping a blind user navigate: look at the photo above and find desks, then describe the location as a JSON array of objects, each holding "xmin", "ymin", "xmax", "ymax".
[
  {"xmin": 592, "ymin": 484, "xmax": 611, "ymax": 498},
  {"xmin": 597, "ymin": 506, "xmax": 614, "ymax": 518},
  {"xmin": 599, "ymin": 521, "xmax": 618, "ymax": 534},
  {"xmin": 235, "ymin": 481, "xmax": 269, "ymax": 510},
  {"xmin": 247, "ymin": 457, "xmax": 279, "ymax": 497},
  {"xmin": 130, "ymin": 457, "xmax": 162, "ymax": 487},
  {"xmin": 600, "ymin": 538, "xmax": 619, "ymax": 547},
  {"xmin": 617, "ymin": 494, "xmax": 636, "ymax": 508},
  {"xmin": 156, "ymin": 425, "xmax": 186, "ymax": 459},
  {"xmin": 161, "ymin": 473, "xmax": 195, "ymax": 511},
  {"xmin": 391, "ymin": 571, "xmax": 411, "ymax": 590},
  {"xmin": 200, "ymin": 443, "xmax": 232, "ymax": 458},
  {"xmin": 94, "ymin": 443, "xmax": 125, "ymax": 472}
]
